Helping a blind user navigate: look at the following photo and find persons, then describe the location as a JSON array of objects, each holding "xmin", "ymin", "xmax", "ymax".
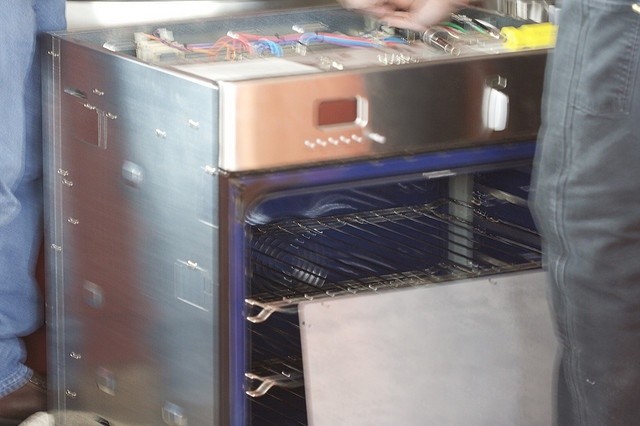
[
  {"xmin": 333, "ymin": 1, "xmax": 639, "ymax": 425},
  {"xmin": 0, "ymin": 0, "xmax": 74, "ymax": 426}
]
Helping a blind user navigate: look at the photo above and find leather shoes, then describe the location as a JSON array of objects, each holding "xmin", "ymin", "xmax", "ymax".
[{"xmin": 1, "ymin": 372, "xmax": 49, "ymax": 423}]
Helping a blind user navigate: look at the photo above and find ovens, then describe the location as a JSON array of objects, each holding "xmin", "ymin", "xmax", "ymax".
[{"xmin": 37, "ymin": 2, "xmax": 572, "ymax": 426}]
